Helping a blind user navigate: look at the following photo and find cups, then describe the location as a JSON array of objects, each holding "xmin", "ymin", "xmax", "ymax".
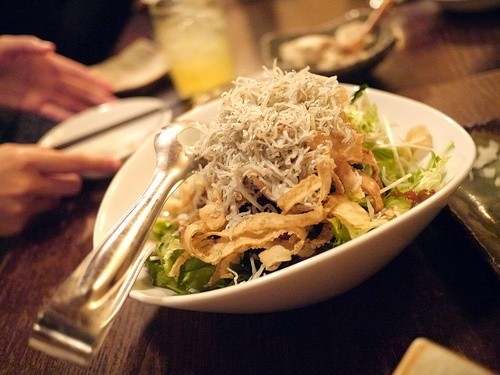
[{"xmin": 146, "ymin": 0, "xmax": 240, "ymax": 101}]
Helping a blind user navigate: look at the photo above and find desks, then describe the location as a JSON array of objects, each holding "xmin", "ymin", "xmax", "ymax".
[{"xmin": 0, "ymin": 0, "xmax": 500, "ymax": 375}]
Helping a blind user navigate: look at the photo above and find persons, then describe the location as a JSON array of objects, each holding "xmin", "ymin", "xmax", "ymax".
[{"xmin": 0, "ymin": 33, "xmax": 122, "ymax": 241}]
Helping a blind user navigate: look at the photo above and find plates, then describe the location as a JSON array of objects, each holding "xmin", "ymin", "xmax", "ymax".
[
  {"xmin": 35, "ymin": 96, "xmax": 173, "ymax": 178},
  {"xmin": 82, "ymin": 37, "xmax": 174, "ymax": 94}
]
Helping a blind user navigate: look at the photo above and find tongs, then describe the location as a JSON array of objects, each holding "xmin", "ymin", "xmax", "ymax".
[{"xmin": 27, "ymin": 118, "xmax": 213, "ymax": 368}]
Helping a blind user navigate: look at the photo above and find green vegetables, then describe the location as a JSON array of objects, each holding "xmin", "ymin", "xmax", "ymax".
[
  {"xmin": 321, "ymin": 82, "xmax": 455, "ymax": 244},
  {"xmin": 144, "ymin": 217, "xmax": 332, "ymax": 296}
]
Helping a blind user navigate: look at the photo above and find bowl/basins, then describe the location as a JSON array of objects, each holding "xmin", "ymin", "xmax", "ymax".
[
  {"xmin": 90, "ymin": 84, "xmax": 477, "ymax": 312},
  {"xmin": 258, "ymin": 7, "xmax": 399, "ymax": 82}
]
